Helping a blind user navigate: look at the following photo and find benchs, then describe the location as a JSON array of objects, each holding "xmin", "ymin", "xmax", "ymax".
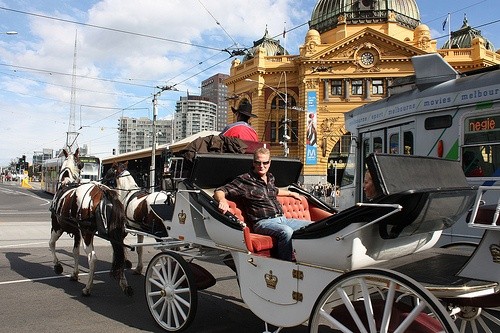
[
  {"xmin": 365, "ymin": 152, "xmax": 483, "ymax": 203},
  {"xmin": 176, "ymin": 151, "xmax": 305, "ymax": 190},
  {"xmin": 218, "ymin": 194, "xmax": 334, "ymax": 253}
]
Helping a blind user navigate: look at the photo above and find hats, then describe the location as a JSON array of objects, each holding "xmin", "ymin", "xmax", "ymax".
[{"xmin": 231, "ymin": 103, "xmax": 257, "ymax": 118}]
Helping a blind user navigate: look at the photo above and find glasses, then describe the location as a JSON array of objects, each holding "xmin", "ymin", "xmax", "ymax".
[{"xmin": 254, "ymin": 161, "xmax": 270, "ymax": 165}]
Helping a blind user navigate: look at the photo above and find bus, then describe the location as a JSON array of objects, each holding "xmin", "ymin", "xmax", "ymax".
[
  {"xmin": 41, "ymin": 155, "xmax": 101, "ymax": 195},
  {"xmin": 336, "ymin": 52, "xmax": 500, "ymax": 284}
]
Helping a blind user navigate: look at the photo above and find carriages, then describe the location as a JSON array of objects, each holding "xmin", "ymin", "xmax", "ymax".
[{"xmin": 47, "ymin": 148, "xmax": 500, "ymax": 333}]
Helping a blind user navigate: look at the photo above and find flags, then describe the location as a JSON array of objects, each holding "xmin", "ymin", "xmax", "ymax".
[
  {"xmin": 442, "ymin": 17, "xmax": 448, "ymax": 31},
  {"xmin": 283, "ymin": 26, "xmax": 286, "ymax": 38}
]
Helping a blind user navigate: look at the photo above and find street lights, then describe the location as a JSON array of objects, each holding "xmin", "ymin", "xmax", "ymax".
[{"xmin": 245, "ymin": 78, "xmax": 289, "ymax": 158}]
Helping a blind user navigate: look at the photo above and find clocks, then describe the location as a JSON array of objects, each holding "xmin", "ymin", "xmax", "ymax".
[{"xmin": 360, "ymin": 52, "xmax": 375, "ymax": 65}]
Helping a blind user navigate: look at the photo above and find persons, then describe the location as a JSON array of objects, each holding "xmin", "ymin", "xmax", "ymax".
[
  {"xmin": 212, "ymin": 148, "xmax": 314, "ymax": 262},
  {"xmin": 363, "ymin": 169, "xmax": 378, "ymax": 203},
  {"xmin": 220, "ymin": 99, "xmax": 259, "ymax": 143}
]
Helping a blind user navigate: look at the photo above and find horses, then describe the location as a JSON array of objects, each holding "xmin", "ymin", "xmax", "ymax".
[
  {"xmin": 100, "ymin": 159, "xmax": 176, "ymax": 278},
  {"xmin": 47, "ymin": 146, "xmax": 136, "ymax": 298}
]
